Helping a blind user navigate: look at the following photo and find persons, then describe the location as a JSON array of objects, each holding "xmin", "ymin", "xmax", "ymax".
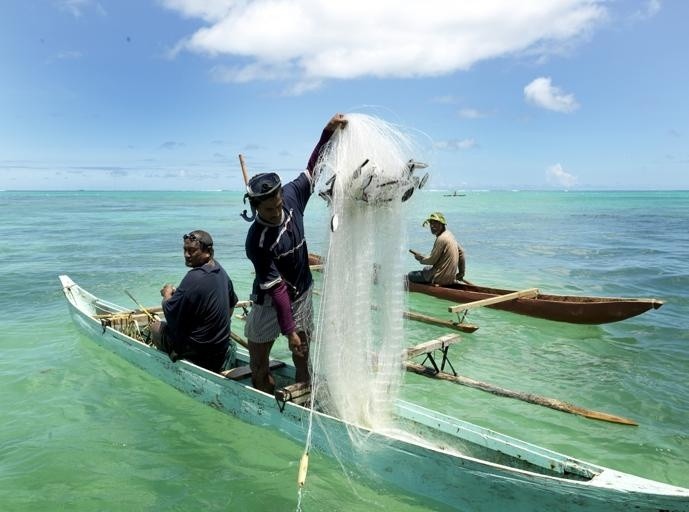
[
  {"xmin": 147, "ymin": 229, "xmax": 239, "ymax": 373},
  {"xmin": 242, "ymin": 113, "xmax": 352, "ymax": 397},
  {"xmin": 405, "ymin": 211, "xmax": 467, "ymax": 286}
]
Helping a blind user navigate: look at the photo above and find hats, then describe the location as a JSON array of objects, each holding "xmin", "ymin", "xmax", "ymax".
[
  {"xmin": 427, "ymin": 211, "xmax": 446, "ymax": 225},
  {"xmin": 246, "ymin": 171, "xmax": 282, "ymax": 201}
]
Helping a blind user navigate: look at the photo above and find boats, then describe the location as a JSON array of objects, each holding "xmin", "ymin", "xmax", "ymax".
[
  {"xmin": 58, "ymin": 272, "xmax": 688, "ymax": 510},
  {"xmin": 306, "ymin": 248, "xmax": 667, "ymax": 325}
]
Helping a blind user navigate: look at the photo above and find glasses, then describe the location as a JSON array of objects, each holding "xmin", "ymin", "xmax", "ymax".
[{"xmin": 182, "ymin": 233, "xmax": 208, "ymax": 247}]
